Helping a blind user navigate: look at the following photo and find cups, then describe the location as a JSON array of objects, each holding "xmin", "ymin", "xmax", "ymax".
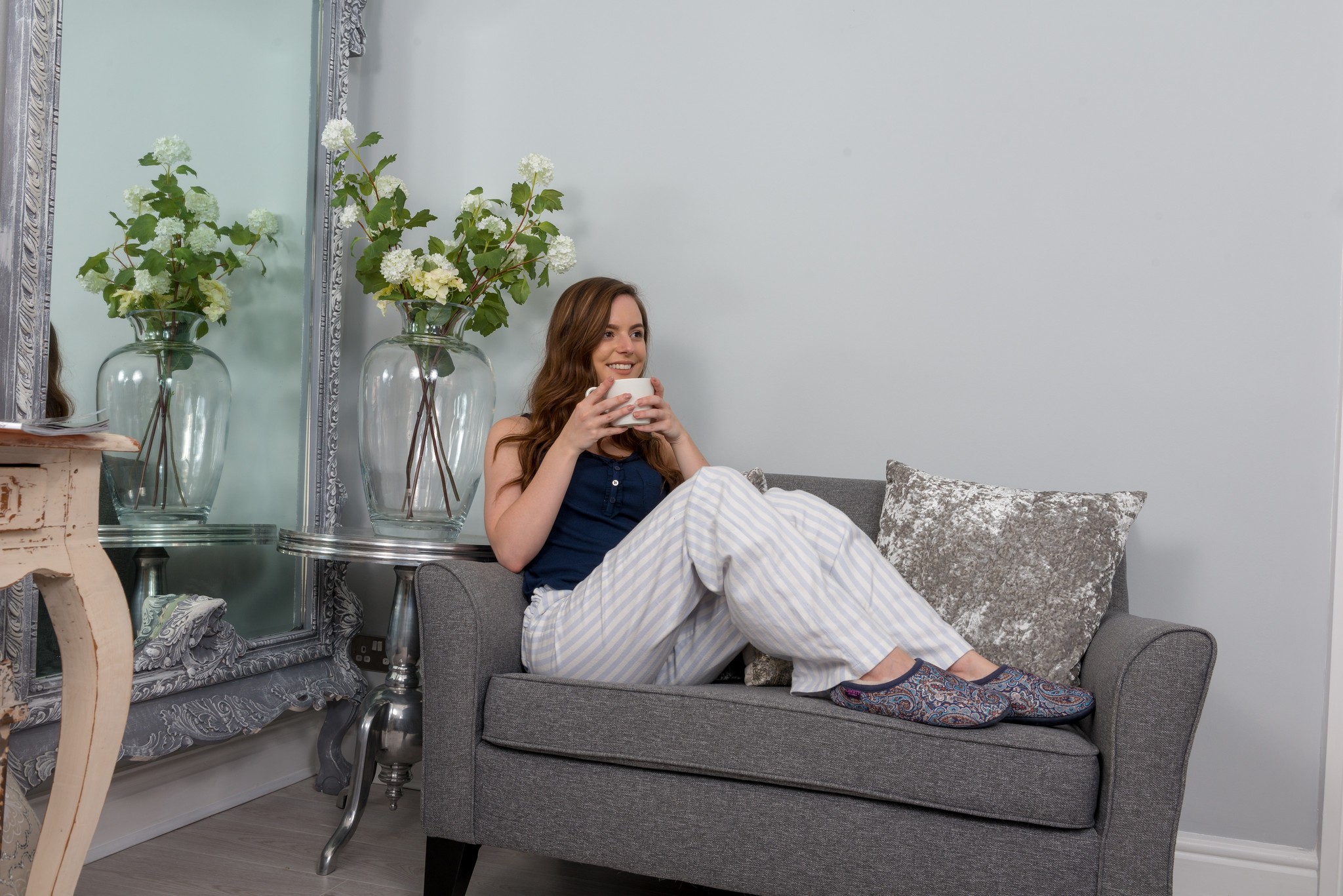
[{"xmin": 585, "ymin": 377, "xmax": 655, "ymax": 428}]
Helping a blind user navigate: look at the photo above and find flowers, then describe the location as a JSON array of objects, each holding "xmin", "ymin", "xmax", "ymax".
[
  {"xmin": 75, "ymin": 133, "xmax": 280, "ymax": 510},
  {"xmin": 320, "ymin": 115, "xmax": 578, "ymax": 520}
]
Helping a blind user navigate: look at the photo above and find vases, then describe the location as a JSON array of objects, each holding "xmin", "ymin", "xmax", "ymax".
[
  {"xmin": 96, "ymin": 310, "xmax": 230, "ymax": 528},
  {"xmin": 355, "ymin": 299, "xmax": 497, "ymax": 542}
]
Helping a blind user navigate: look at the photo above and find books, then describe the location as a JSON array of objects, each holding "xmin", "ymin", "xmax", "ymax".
[{"xmin": 0, "ymin": 408, "xmax": 110, "ymax": 436}]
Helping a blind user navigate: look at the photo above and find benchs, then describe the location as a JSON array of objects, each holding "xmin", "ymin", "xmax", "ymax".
[{"xmin": 410, "ymin": 473, "xmax": 1219, "ymax": 896}]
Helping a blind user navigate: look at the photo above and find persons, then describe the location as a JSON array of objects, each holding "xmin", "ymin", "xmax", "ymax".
[
  {"xmin": 46, "ymin": 320, "xmax": 76, "ymax": 417},
  {"xmin": 484, "ymin": 276, "xmax": 1096, "ymax": 730}
]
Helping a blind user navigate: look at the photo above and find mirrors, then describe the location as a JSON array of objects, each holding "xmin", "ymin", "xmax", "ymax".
[{"xmin": 0, "ymin": 0, "xmax": 374, "ymax": 798}]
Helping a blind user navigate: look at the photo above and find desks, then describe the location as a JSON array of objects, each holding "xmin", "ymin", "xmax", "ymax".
[
  {"xmin": 96, "ymin": 521, "xmax": 278, "ymax": 641},
  {"xmin": 0, "ymin": 431, "xmax": 140, "ymax": 896},
  {"xmin": 276, "ymin": 526, "xmax": 497, "ymax": 875}
]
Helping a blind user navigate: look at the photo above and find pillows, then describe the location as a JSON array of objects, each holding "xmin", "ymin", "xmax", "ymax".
[
  {"xmin": 877, "ymin": 457, "xmax": 1147, "ymax": 687},
  {"xmin": 739, "ymin": 465, "xmax": 792, "ymax": 687}
]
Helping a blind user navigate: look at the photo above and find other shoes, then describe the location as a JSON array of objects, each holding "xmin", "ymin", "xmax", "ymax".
[
  {"xmin": 970, "ymin": 663, "xmax": 1093, "ymax": 723},
  {"xmin": 830, "ymin": 656, "xmax": 1011, "ymax": 729}
]
